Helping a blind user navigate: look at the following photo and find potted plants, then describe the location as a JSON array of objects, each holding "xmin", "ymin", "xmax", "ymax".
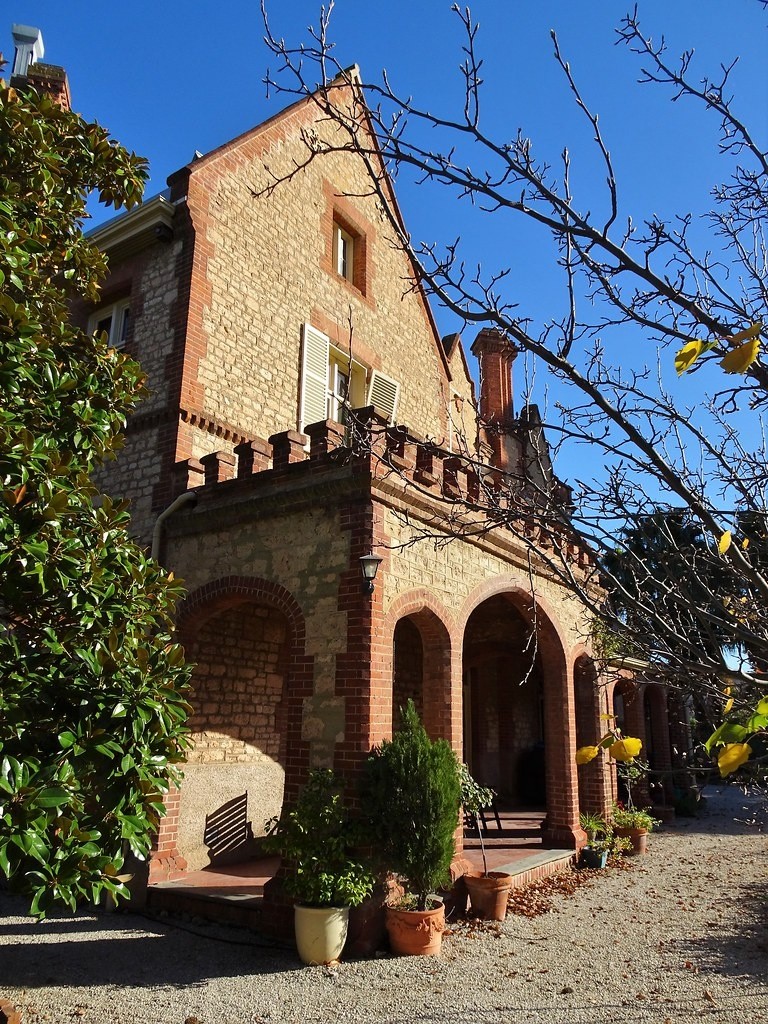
[
  {"xmin": 607, "ymin": 804, "xmax": 661, "ymax": 854},
  {"xmin": 356, "ymin": 697, "xmax": 463, "ymax": 954},
  {"xmin": 458, "ymin": 764, "xmax": 512, "ymax": 920},
  {"xmin": 584, "ymin": 840, "xmax": 611, "ymax": 869},
  {"xmin": 580, "ymin": 810, "xmax": 609, "ymax": 842},
  {"xmin": 261, "ymin": 770, "xmax": 376, "ymax": 966}
]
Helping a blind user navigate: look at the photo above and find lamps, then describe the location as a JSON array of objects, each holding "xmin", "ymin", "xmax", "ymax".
[{"xmin": 357, "ymin": 551, "xmax": 383, "ymax": 594}]
